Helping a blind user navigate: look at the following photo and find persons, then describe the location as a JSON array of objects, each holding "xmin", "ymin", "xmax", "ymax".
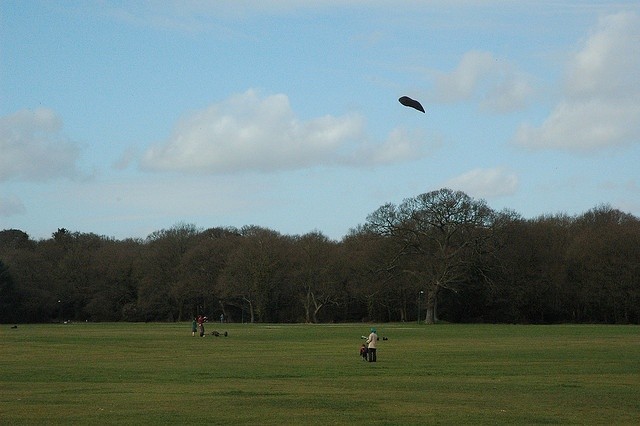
[
  {"xmin": 198, "ymin": 315, "xmax": 206, "ymax": 337},
  {"xmin": 220, "ymin": 313, "xmax": 224, "ymax": 323},
  {"xmin": 192, "ymin": 317, "xmax": 197, "ymax": 337},
  {"xmin": 366, "ymin": 329, "xmax": 377, "ymax": 362},
  {"xmin": 359, "ymin": 345, "xmax": 369, "ymax": 362}
]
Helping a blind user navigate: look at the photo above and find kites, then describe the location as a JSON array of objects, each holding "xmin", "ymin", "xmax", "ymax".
[{"xmin": 399, "ymin": 96, "xmax": 425, "ymax": 113}]
